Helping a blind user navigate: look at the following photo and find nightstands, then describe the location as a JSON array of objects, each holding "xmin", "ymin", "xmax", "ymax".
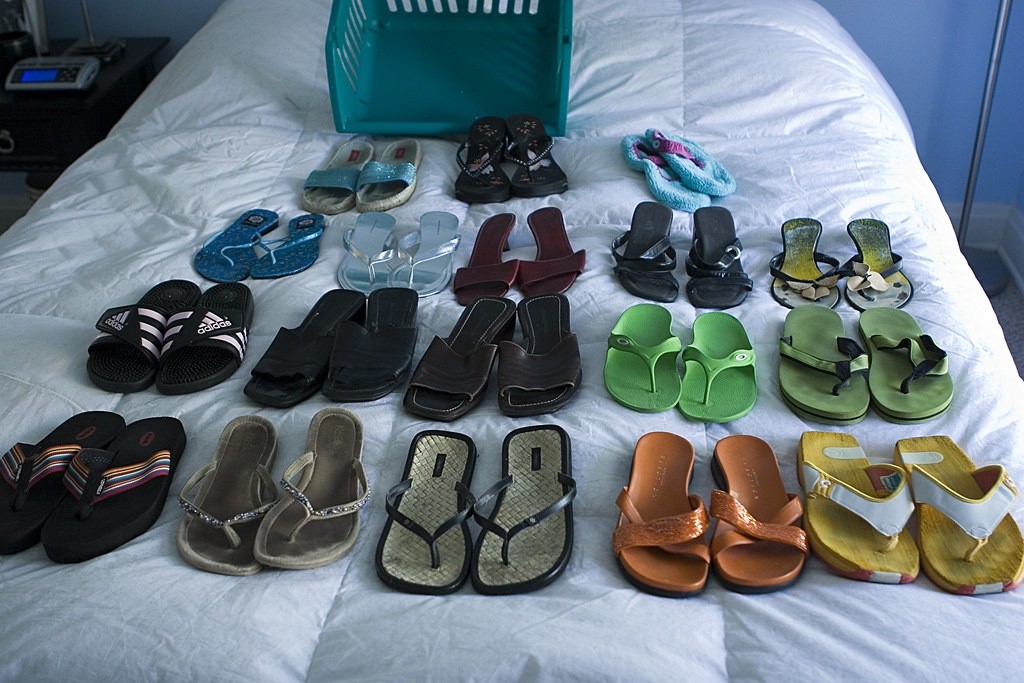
[{"xmin": 0, "ymin": 37, "xmax": 170, "ymax": 205}]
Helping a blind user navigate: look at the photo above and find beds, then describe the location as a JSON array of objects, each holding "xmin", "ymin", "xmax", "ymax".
[{"xmin": 0, "ymin": 0, "xmax": 1024, "ymax": 683}]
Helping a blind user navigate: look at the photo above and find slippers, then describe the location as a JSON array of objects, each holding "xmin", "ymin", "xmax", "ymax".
[
  {"xmin": 337, "ymin": 210, "xmax": 393, "ymax": 298},
  {"xmin": 497, "ymin": 292, "xmax": 583, "ymax": 417},
  {"xmin": 468, "ymin": 423, "xmax": 578, "ymax": 597},
  {"xmin": 515, "ymin": 206, "xmax": 586, "ymax": 299},
  {"xmin": 679, "ymin": 311, "xmax": 758, "ymax": 425},
  {"xmin": 319, "ymin": 287, "xmax": 420, "ymax": 402},
  {"xmin": 684, "ymin": 205, "xmax": 754, "ymax": 309},
  {"xmin": 610, "ymin": 429, "xmax": 711, "ymax": 600},
  {"xmin": 894, "ymin": 433, "xmax": 1024, "ymax": 597},
  {"xmin": 372, "ymin": 428, "xmax": 478, "ymax": 597},
  {"xmin": 387, "ymin": 210, "xmax": 463, "ymax": 298},
  {"xmin": 40, "ymin": 414, "xmax": 188, "ymax": 564},
  {"xmin": 795, "ymin": 429, "xmax": 921, "ymax": 585},
  {"xmin": 767, "ymin": 217, "xmax": 842, "ymax": 312},
  {"xmin": 301, "ymin": 139, "xmax": 374, "ymax": 215},
  {"xmin": 454, "ymin": 115, "xmax": 510, "ymax": 203},
  {"xmin": 776, "ymin": 304, "xmax": 871, "ymax": 426},
  {"xmin": 193, "ymin": 207, "xmax": 280, "ymax": 282},
  {"xmin": 156, "ymin": 280, "xmax": 256, "ymax": 395},
  {"xmin": 838, "ymin": 218, "xmax": 915, "ymax": 313},
  {"xmin": 505, "ymin": 114, "xmax": 569, "ymax": 198},
  {"xmin": 85, "ymin": 278, "xmax": 202, "ymax": 394},
  {"xmin": 175, "ymin": 414, "xmax": 277, "ymax": 578},
  {"xmin": 253, "ymin": 405, "xmax": 371, "ymax": 571},
  {"xmin": 0, "ymin": 409, "xmax": 126, "ymax": 558},
  {"xmin": 603, "ymin": 303, "xmax": 683, "ymax": 413},
  {"xmin": 857, "ymin": 305, "xmax": 954, "ymax": 425},
  {"xmin": 453, "ymin": 212, "xmax": 519, "ymax": 306},
  {"xmin": 709, "ymin": 433, "xmax": 808, "ymax": 597},
  {"xmin": 403, "ymin": 294, "xmax": 518, "ymax": 423},
  {"xmin": 249, "ymin": 212, "xmax": 326, "ymax": 280},
  {"xmin": 243, "ymin": 289, "xmax": 367, "ymax": 410},
  {"xmin": 609, "ymin": 201, "xmax": 681, "ymax": 302},
  {"xmin": 354, "ymin": 138, "xmax": 423, "ymax": 212}
]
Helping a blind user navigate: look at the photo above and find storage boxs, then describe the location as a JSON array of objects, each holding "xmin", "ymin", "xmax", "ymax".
[{"xmin": 324, "ymin": 0, "xmax": 574, "ymax": 139}]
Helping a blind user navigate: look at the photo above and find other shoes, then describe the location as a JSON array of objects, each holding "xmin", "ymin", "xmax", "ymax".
[
  {"xmin": 645, "ymin": 125, "xmax": 736, "ymax": 196},
  {"xmin": 620, "ymin": 133, "xmax": 712, "ymax": 212}
]
